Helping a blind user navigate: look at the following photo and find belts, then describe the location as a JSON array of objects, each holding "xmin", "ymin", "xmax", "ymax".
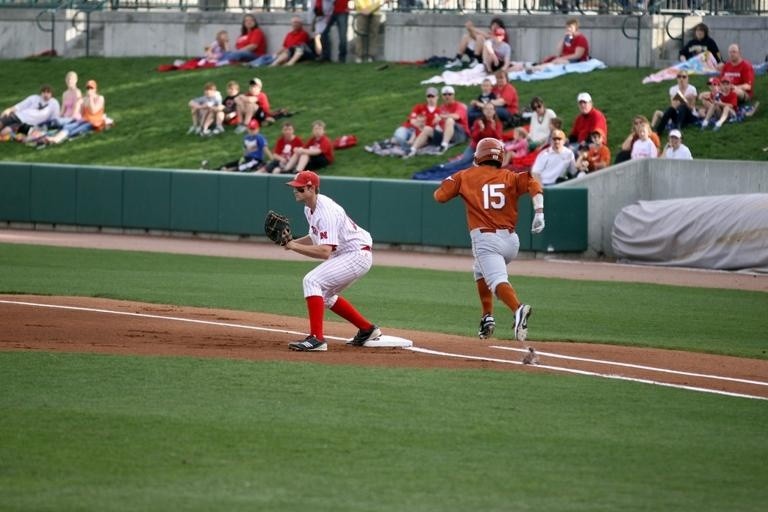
[
  {"xmin": 480, "ymin": 226, "xmax": 514, "ymax": 234},
  {"xmin": 364, "ymin": 247, "xmax": 374, "ymax": 253}
]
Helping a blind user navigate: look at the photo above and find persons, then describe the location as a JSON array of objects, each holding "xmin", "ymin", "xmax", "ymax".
[
  {"xmin": 185, "ymin": 81, "xmax": 222, "ymax": 136},
  {"xmin": 444, "ymin": 18, "xmax": 523, "ymax": 75},
  {"xmin": 394, "ymin": 70, "xmax": 611, "ymax": 185},
  {"xmin": 256, "ymin": 121, "xmax": 304, "ymax": 174},
  {"xmin": 200, "ymin": 118, "xmax": 272, "ymax": 172},
  {"xmin": 614, "ymin": 114, "xmax": 661, "ymax": 164},
  {"xmin": 216, "ymin": 14, "xmax": 265, "ymax": 64},
  {"xmin": 431, "ymin": 137, "xmax": 545, "ymax": 342},
  {"xmin": 678, "ymin": 23, "xmax": 726, "ymax": 70},
  {"xmin": 697, "ymin": 43, "xmax": 752, "ymax": 132},
  {"xmin": 199, "ymin": 80, "xmax": 240, "ymax": 135},
  {"xmin": 41, "ymin": 79, "xmax": 104, "ymax": 143},
  {"xmin": 37, "ymin": 70, "xmax": 82, "ymax": 137},
  {"xmin": 0, "ymin": 82, "xmax": 59, "ymax": 131},
  {"xmin": 525, "ymin": 18, "xmax": 590, "ymax": 74},
  {"xmin": 352, "ymin": 0, "xmax": 386, "ymax": 63},
  {"xmin": 196, "ymin": 30, "xmax": 231, "ymax": 68},
  {"xmin": 268, "ymin": 15, "xmax": 315, "ymax": 70},
  {"xmin": 281, "ymin": 170, "xmax": 382, "ymax": 353},
  {"xmin": 631, "ymin": 123, "xmax": 658, "ymax": 160},
  {"xmin": 281, "ymin": 119, "xmax": 334, "ymax": 175},
  {"xmin": 651, "ymin": 69, "xmax": 699, "ymax": 137},
  {"xmin": 102, "ymin": 1, "xmax": 755, "ymax": 16},
  {"xmin": 660, "ymin": 129, "xmax": 693, "ymax": 160},
  {"xmin": 314, "ymin": 0, "xmax": 349, "ymax": 64},
  {"xmin": 233, "ymin": 77, "xmax": 274, "ymax": 135}
]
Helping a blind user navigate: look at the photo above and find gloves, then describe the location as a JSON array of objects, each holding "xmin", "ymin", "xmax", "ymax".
[{"xmin": 531, "ymin": 213, "xmax": 545, "ymax": 234}]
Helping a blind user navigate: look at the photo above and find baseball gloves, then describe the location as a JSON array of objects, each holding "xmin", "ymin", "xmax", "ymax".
[{"xmin": 265, "ymin": 211, "xmax": 293, "ymax": 245}]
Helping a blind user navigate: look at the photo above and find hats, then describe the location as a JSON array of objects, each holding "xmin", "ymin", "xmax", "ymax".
[
  {"xmin": 247, "ymin": 120, "xmax": 258, "ymax": 128},
  {"xmin": 286, "ymin": 171, "xmax": 321, "ymax": 188},
  {"xmin": 591, "ymin": 128, "xmax": 604, "ymax": 135},
  {"xmin": 249, "ymin": 76, "xmax": 263, "ymax": 88},
  {"xmin": 577, "ymin": 93, "xmax": 592, "ymax": 103},
  {"xmin": 441, "ymin": 86, "xmax": 455, "ymax": 94},
  {"xmin": 495, "ymin": 28, "xmax": 504, "ymax": 36},
  {"xmin": 85, "ymin": 80, "xmax": 98, "ymax": 89},
  {"xmin": 668, "ymin": 129, "xmax": 681, "ymax": 139},
  {"xmin": 708, "ymin": 77, "xmax": 718, "ymax": 83},
  {"xmin": 720, "ymin": 76, "xmax": 732, "ymax": 83},
  {"xmin": 425, "ymin": 88, "xmax": 438, "ymax": 96}
]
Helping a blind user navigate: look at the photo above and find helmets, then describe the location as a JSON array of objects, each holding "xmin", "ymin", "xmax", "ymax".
[{"xmin": 475, "ymin": 137, "xmax": 503, "ymax": 168}]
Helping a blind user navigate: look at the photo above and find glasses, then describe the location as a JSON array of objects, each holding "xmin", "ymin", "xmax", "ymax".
[
  {"xmin": 296, "ymin": 187, "xmax": 309, "ymax": 193},
  {"xmin": 532, "ymin": 104, "xmax": 542, "ymax": 110},
  {"xmin": 677, "ymin": 75, "xmax": 688, "ymax": 79},
  {"xmin": 444, "ymin": 93, "xmax": 452, "ymax": 96},
  {"xmin": 551, "ymin": 137, "xmax": 561, "ymax": 141},
  {"xmin": 427, "ymin": 95, "xmax": 435, "ymax": 98}
]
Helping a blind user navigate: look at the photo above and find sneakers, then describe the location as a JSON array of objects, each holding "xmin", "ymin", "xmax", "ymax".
[
  {"xmin": 188, "ymin": 124, "xmax": 246, "ymax": 137},
  {"xmin": 468, "ymin": 57, "xmax": 479, "ymax": 68},
  {"xmin": 712, "ymin": 122, "xmax": 721, "ymax": 131},
  {"xmin": 513, "ymin": 305, "xmax": 532, "ymax": 341},
  {"xmin": 287, "ymin": 337, "xmax": 328, "ymax": 353},
  {"xmin": 444, "ymin": 58, "xmax": 462, "ymax": 68},
  {"xmin": 477, "ymin": 314, "xmax": 494, "ymax": 339},
  {"xmin": 699, "ymin": 120, "xmax": 708, "ymax": 129},
  {"xmin": 347, "ymin": 325, "xmax": 382, "ymax": 346}
]
